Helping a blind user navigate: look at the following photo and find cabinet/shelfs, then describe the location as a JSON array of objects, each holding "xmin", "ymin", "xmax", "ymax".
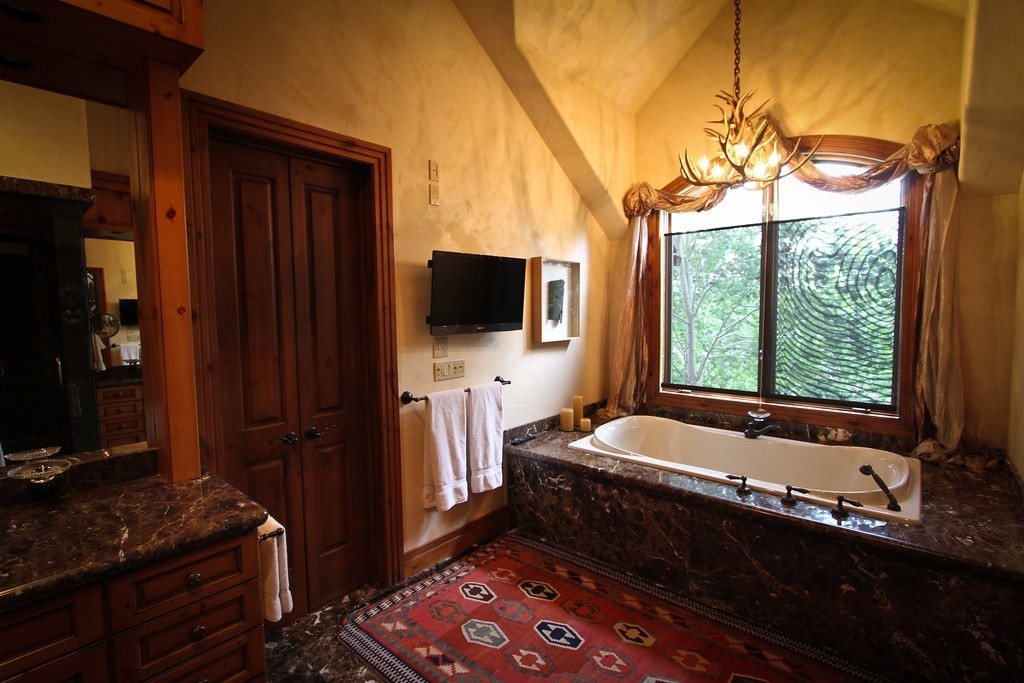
[
  {"xmin": 95, "ymin": 384, "xmax": 149, "ymax": 449},
  {"xmin": 0, "ymin": 529, "xmax": 267, "ymax": 682},
  {"xmin": 0, "ymin": 199, "xmax": 99, "ymax": 467}
]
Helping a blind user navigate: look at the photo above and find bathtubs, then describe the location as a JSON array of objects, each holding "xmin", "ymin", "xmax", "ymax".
[{"xmin": 566, "ymin": 414, "xmax": 925, "ymax": 528}]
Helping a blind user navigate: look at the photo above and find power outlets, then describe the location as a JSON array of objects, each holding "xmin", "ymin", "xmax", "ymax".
[{"xmin": 432, "ymin": 337, "xmax": 448, "ymax": 358}]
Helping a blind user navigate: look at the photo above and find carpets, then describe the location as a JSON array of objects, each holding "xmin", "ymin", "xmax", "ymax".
[{"xmin": 336, "ymin": 536, "xmax": 889, "ymax": 683}]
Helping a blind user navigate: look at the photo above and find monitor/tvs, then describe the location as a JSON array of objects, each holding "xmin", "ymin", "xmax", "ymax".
[
  {"xmin": 119, "ymin": 299, "xmax": 140, "ymax": 326},
  {"xmin": 429, "ymin": 251, "xmax": 526, "ymax": 336}
]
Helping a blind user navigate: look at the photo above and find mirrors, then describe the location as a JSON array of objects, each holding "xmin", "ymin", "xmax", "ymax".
[{"xmin": 82, "ymin": 238, "xmax": 149, "ymax": 450}]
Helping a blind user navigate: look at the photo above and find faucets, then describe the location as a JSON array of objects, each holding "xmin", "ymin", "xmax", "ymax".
[{"xmin": 859, "ymin": 463, "xmax": 902, "ymax": 513}]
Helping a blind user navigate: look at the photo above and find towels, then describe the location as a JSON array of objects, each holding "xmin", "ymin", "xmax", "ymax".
[
  {"xmin": 422, "ymin": 387, "xmax": 469, "ymax": 512},
  {"xmin": 468, "ymin": 379, "xmax": 503, "ymax": 494},
  {"xmin": 119, "ymin": 343, "xmax": 141, "ymax": 365},
  {"xmin": 92, "ymin": 333, "xmax": 106, "ymax": 373},
  {"xmin": 258, "ymin": 514, "xmax": 294, "ymax": 624}
]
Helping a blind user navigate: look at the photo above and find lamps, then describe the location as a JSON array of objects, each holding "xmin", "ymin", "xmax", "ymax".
[{"xmin": 674, "ymin": 0, "xmax": 827, "ymax": 194}]
